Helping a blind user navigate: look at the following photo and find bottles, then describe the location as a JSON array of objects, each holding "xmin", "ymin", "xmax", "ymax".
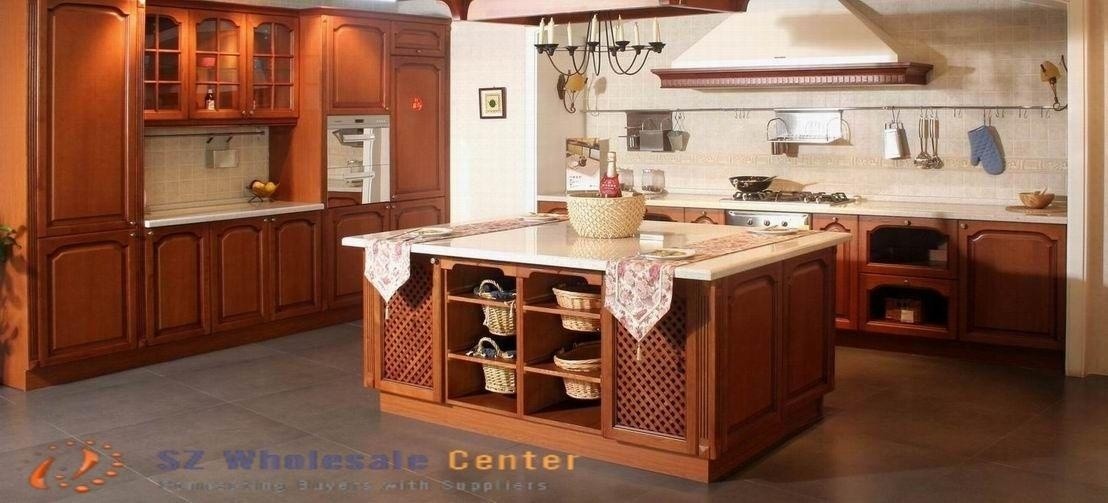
[
  {"xmin": 204, "ymin": 89, "xmax": 216, "ymax": 111},
  {"xmin": 600, "ymin": 151, "xmax": 622, "ymax": 199},
  {"xmin": 640, "ymin": 169, "xmax": 655, "ymax": 191}
]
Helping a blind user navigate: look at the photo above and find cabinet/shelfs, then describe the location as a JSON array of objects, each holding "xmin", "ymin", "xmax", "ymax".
[
  {"xmin": 392, "ymin": 14, "xmax": 454, "ymax": 199},
  {"xmin": 145, "ymin": 3, "xmax": 300, "ymax": 126},
  {"xmin": 713, "ymin": 243, "xmax": 846, "ymax": 489},
  {"xmin": 302, "ymin": 12, "xmax": 391, "ymax": 114},
  {"xmin": 814, "ymin": 211, "xmax": 1069, "ymax": 357},
  {"xmin": 145, "ymin": 212, "xmax": 326, "ymax": 368},
  {"xmin": 322, "ymin": 198, "xmax": 458, "ymax": 328}
]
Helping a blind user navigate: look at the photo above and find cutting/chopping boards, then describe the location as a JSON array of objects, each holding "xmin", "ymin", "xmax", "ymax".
[{"xmin": 1004, "ymin": 205, "xmax": 1068, "ymax": 215}]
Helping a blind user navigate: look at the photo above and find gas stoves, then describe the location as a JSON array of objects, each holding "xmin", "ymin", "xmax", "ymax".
[{"xmin": 734, "ymin": 188, "xmax": 851, "ymax": 204}]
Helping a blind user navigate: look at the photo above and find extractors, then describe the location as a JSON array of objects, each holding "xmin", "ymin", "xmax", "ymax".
[{"xmin": 650, "ymin": 61, "xmax": 934, "ymax": 88}]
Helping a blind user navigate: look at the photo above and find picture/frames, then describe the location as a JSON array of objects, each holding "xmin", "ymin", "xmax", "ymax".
[{"xmin": 477, "ymin": 86, "xmax": 507, "ymax": 119}]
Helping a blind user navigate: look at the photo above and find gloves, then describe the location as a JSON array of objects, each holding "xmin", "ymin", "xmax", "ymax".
[{"xmin": 968, "ymin": 125, "xmax": 1004, "ymax": 174}]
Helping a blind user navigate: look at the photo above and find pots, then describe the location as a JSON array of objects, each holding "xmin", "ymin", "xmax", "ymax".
[{"xmin": 729, "ymin": 175, "xmax": 774, "ymax": 191}]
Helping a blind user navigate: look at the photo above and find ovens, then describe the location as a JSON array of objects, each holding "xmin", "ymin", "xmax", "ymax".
[{"xmin": 725, "ymin": 210, "xmax": 812, "ymax": 228}]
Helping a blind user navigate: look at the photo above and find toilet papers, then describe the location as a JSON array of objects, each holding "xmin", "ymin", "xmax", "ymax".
[
  {"xmin": 344, "ymin": 172, "xmax": 375, "ymax": 179},
  {"xmin": 343, "ymin": 133, "xmax": 376, "ymax": 143}
]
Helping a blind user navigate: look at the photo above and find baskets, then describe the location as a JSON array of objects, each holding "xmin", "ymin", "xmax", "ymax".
[
  {"xmin": 474, "ymin": 279, "xmax": 516, "ymax": 337},
  {"xmin": 552, "ymin": 287, "xmax": 601, "ymax": 332},
  {"xmin": 554, "ymin": 340, "xmax": 601, "ymax": 400},
  {"xmin": 567, "ymin": 191, "xmax": 646, "ymax": 239},
  {"xmin": 473, "ymin": 337, "xmax": 517, "ymax": 394}
]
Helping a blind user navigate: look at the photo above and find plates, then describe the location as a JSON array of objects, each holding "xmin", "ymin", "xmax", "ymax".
[
  {"xmin": 647, "ymin": 247, "xmax": 696, "ymax": 259},
  {"xmin": 520, "ymin": 213, "xmax": 557, "ymax": 222},
  {"xmin": 749, "ymin": 226, "xmax": 800, "ymax": 236},
  {"xmin": 408, "ymin": 228, "xmax": 457, "ymax": 237}
]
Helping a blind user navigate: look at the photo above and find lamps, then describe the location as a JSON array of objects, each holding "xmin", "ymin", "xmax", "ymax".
[{"xmin": 533, "ymin": 10, "xmax": 666, "ymax": 117}]
